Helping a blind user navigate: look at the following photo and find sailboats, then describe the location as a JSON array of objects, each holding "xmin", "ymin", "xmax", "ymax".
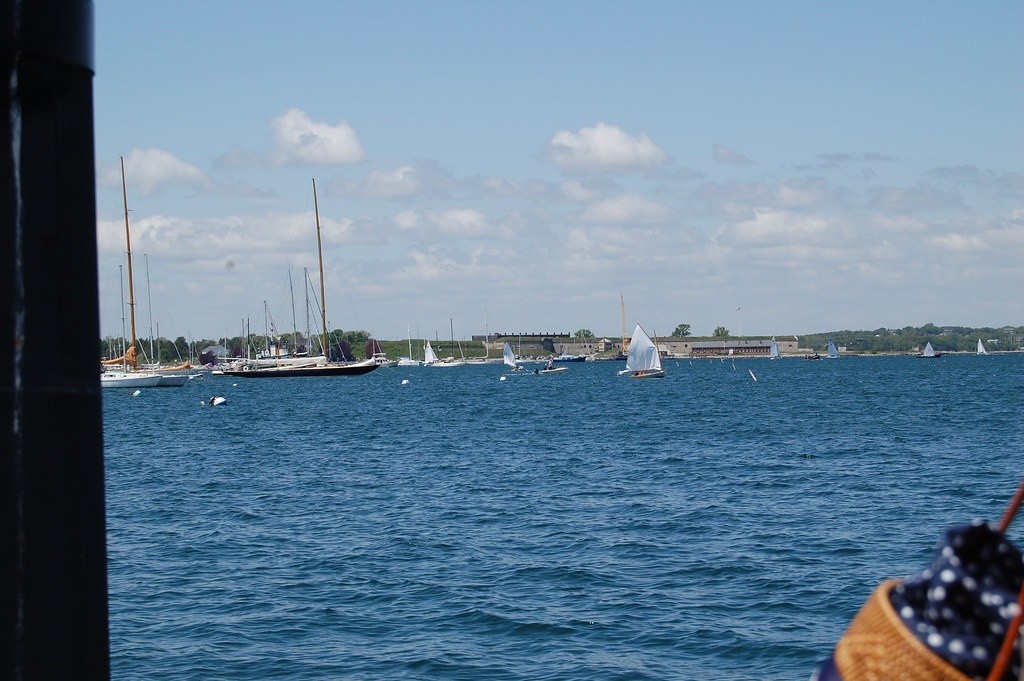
[
  {"xmin": 825, "ymin": 337, "xmax": 842, "ymax": 359},
  {"xmin": 101, "ymin": 156, "xmax": 587, "ymax": 389},
  {"xmin": 768, "ymin": 335, "xmax": 781, "ymax": 360},
  {"xmin": 914, "ymin": 342, "xmax": 935, "ymax": 358},
  {"xmin": 617, "ymin": 323, "xmax": 666, "ymax": 379},
  {"xmin": 976, "ymin": 338, "xmax": 989, "ymax": 356}
]
